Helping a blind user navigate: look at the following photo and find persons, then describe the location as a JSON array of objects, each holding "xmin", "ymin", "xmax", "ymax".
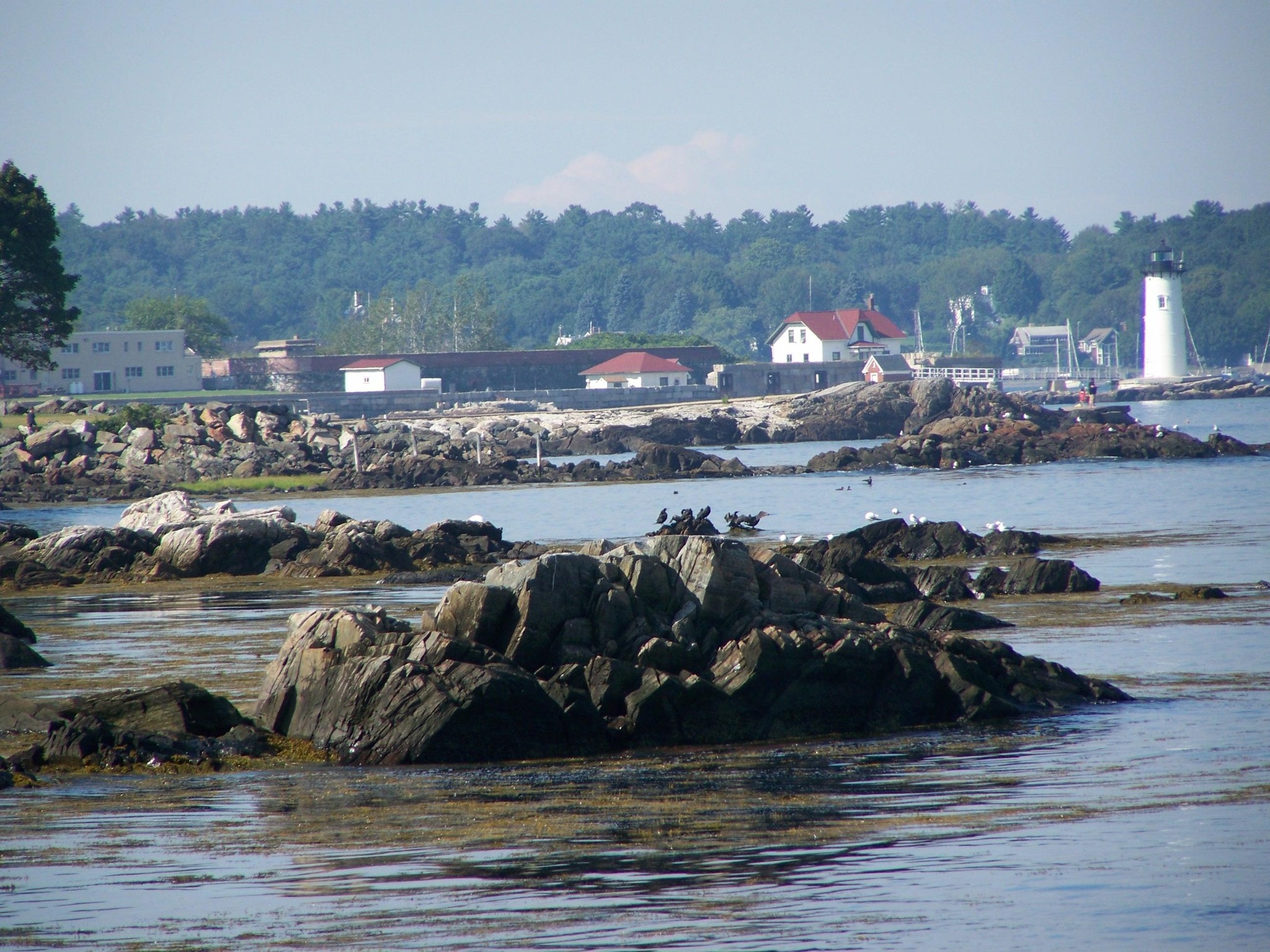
[
  {"xmin": 26, "ymin": 407, "xmax": 37, "ymax": 435},
  {"xmin": 1078, "ymin": 387, "xmax": 1087, "ymax": 409},
  {"xmin": 1088, "ymin": 377, "xmax": 1099, "ymax": 408}
]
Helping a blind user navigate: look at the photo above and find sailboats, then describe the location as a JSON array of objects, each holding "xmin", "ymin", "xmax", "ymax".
[{"xmin": 1055, "ymin": 307, "xmax": 1270, "ymax": 392}]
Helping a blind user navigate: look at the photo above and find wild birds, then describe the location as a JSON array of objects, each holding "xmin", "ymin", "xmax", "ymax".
[
  {"xmin": 1135, "ymin": 418, "xmax": 1143, "ymax": 423},
  {"xmin": 847, "ymin": 486, "xmax": 852, "ymax": 491},
  {"xmin": 1023, "ymin": 413, "xmax": 1032, "ymax": 420},
  {"xmin": 900, "ymin": 430, "xmax": 904, "ymax": 437},
  {"xmin": 985, "ymin": 424, "xmax": 993, "ymax": 433},
  {"xmin": 1076, "ymin": 417, "xmax": 1081, "ymax": 423},
  {"xmin": 1002, "ymin": 412, "xmax": 1012, "ymax": 417},
  {"xmin": 779, "ymin": 534, "xmax": 834, "ymax": 546},
  {"xmin": 862, "ymin": 476, "xmax": 872, "ymax": 485},
  {"xmin": 653, "ymin": 506, "xmax": 769, "ymax": 529},
  {"xmin": 1107, "ymin": 425, "xmax": 1223, "ymax": 437},
  {"xmin": 865, "ymin": 508, "xmax": 1017, "ymax": 532},
  {"xmin": 835, "ymin": 487, "xmax": 844, "ymax": 490}
]
